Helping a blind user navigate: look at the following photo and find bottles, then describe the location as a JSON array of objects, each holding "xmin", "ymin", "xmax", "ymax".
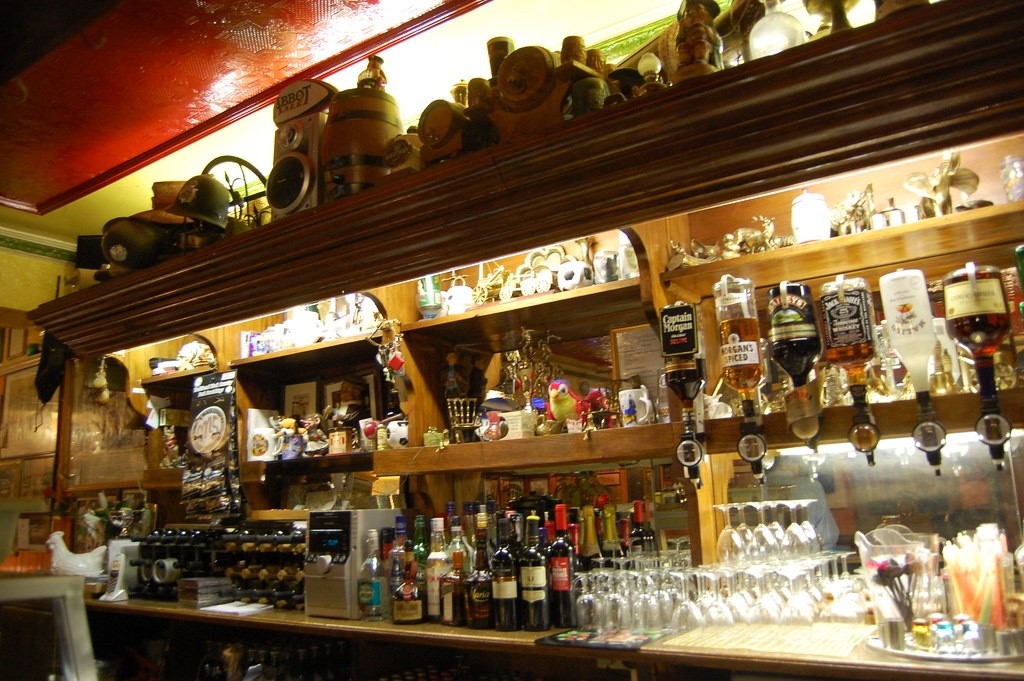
[
  {"xmin": 657, "ymin": 243, "xmax": 1024, "ymax": 486},
  {"xmin": 416, "ymin": 275, "xmax": 442, "ymax": 320},
  {"xmin": 127, "ymin": 524, "xmax": 305, "ymax": 611},
  {"xmin": 1000, "ymin": 154, "xmax": 1024, "ymax": 203},
  {"xmin": 357, "ymin": 495, "xmax": 659, "ymax": 632},
  {"xmin": 791, "ymin": 188, "xmax": 830, "ymax": 242}
]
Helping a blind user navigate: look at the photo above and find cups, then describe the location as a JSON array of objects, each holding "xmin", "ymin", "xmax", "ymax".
[
  {"xmin": 855, "ymin": 523, "xmax": 1024, "ymax": 659},
  {"xmin": 291, "ymin": 310, "xmax": 324, "ymax": 349},
  {"xmin": 247, "ymin": 427, "xmax": 284, "ymax": 462}
]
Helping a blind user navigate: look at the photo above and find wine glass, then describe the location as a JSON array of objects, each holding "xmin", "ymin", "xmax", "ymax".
[{"xmin": 569, "ymin": 498, "xmax": 874, "ymax": 637}]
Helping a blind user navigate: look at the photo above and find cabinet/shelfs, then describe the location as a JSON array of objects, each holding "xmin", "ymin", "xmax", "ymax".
[{"xmin": 25, "ymin": 0, "xmax": 1024, "ymax": 681}]
